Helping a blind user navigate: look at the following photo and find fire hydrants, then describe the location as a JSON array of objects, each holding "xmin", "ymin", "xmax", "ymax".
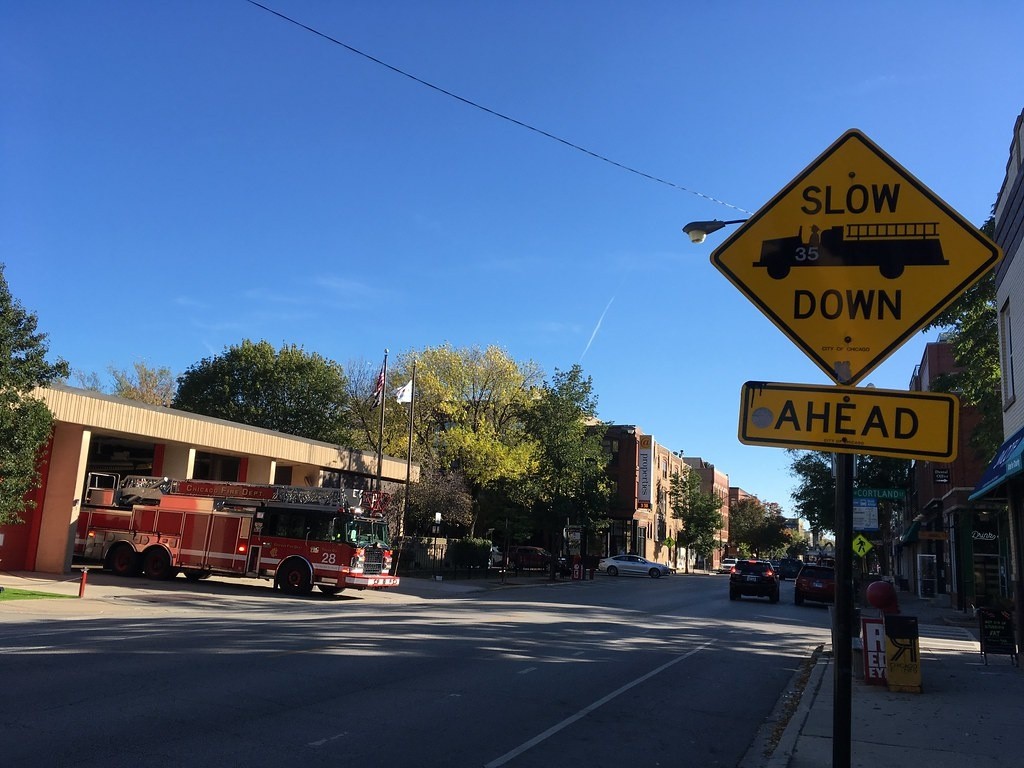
[{"xmin": 588, "ymin": 565, "xmax": 596, "ymax": 578}]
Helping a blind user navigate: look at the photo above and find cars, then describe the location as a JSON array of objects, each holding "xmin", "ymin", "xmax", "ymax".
[
  {"xmin": 599, "ymin": 554, "xmax": 668, "ymax": 579},
  {"xmin": 766, "ymin": 557, "xmax": 834, "ymax": 606}
]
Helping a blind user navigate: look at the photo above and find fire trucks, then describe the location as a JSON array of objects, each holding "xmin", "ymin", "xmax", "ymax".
[{"xmin": 73, "ymin": 472, "xmax": 400, "ymax": 598}]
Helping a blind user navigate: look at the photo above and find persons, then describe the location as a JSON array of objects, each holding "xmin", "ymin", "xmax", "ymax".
[{"xmin": 589, "ymin": 556, "xmax": 595, "ymax": 580}]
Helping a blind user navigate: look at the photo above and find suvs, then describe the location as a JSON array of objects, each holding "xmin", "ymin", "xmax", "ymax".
[
  {"xmin": 719, "ymin": 558, "xmax": 738, "ymax": 574},
  {"xmin": 729, "ymin": 558, "xmax": 780, "ymax": 604},
  {"xmin": 491, "ymin": 546, "xmax": 563, "ymax": 573}
]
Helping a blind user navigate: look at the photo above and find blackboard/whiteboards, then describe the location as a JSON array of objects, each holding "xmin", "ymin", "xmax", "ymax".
[{"xmin": 979, "ymin": 607, "xmax": 1018, "ymax": 655}]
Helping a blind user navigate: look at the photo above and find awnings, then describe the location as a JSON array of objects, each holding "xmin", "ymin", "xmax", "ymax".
[
  {"xmin": 901, "ymin": 521, "xmax": 921, "ymax": 547},
  {"xmin": 966, "ymin": 424, "xmax": 1024, "ymax": 506}
]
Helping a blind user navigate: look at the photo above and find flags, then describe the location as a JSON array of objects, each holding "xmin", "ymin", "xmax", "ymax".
[
  {"xmin": 369, "ymin": 360, "xmax": 385, "ymax": 413},
  {"xmin": 396, "ymin": 379, "xmax": 413, "ymax": 405}
]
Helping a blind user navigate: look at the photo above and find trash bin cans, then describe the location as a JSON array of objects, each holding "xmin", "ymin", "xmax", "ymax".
[
  {"xmin": 828, "ymin": 605, "xmax": 862, "ymax": 658},
  {"xmin": 572, "ymin": 554, "xmax": 584, "ymax": 581}
]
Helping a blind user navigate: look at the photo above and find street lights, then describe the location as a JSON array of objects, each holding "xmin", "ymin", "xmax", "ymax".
[{"xmin": 681, "ymin": 220, "xmax": 852, "ymax": 767}]
